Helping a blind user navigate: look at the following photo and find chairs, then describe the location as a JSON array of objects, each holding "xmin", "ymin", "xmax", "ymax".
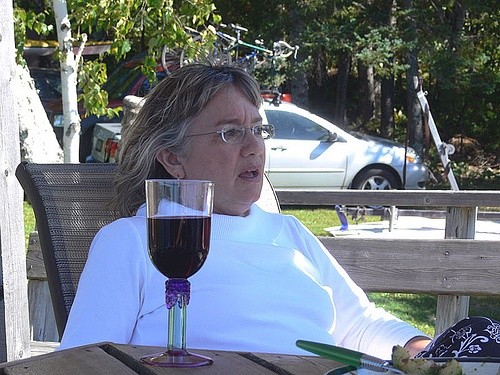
[{"xmin": 14, "ymin": 159, "xmax": 282, "ymax": 343}]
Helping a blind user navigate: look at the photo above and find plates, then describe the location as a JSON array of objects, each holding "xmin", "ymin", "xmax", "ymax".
[{"xmin": 322, "ymin": 359, "xmax": 500, "ymax": 375}]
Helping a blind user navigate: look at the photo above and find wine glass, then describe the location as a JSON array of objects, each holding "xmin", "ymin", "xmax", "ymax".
[{"xmin": 139, "ymin": 179, "xmax": 213, "ymax": 367}]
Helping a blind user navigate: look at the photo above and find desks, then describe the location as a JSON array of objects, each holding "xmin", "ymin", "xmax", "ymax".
[{"xmin": 0, "ymin": 342, "xmax": 393, "ymax": 375}]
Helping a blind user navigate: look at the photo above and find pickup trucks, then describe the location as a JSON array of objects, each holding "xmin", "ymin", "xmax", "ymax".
[{"xmin": 22, "ymin": 40, "xmax": 293, "ymax": 142}]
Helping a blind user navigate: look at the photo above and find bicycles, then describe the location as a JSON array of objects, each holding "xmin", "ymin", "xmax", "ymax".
[{"xmin": 161, "ymin": 22, "xmax": 299, "ymax": 77}]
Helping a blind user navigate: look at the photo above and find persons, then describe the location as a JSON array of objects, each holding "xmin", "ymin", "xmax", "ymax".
[{"xmin": 56, "ymin": 64, "xmax": 433, "ymax": 362}]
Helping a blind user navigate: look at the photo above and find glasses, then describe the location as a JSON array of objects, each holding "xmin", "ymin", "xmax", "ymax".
[{"xmin": 175, "ymin": 123, "xmax": 276, "ymax": 145}]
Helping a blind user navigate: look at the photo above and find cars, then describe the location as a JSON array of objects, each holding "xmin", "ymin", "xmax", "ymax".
[{"xmin": 78, "ymin": 99, "xmax": 428, "ymax": 208}]
